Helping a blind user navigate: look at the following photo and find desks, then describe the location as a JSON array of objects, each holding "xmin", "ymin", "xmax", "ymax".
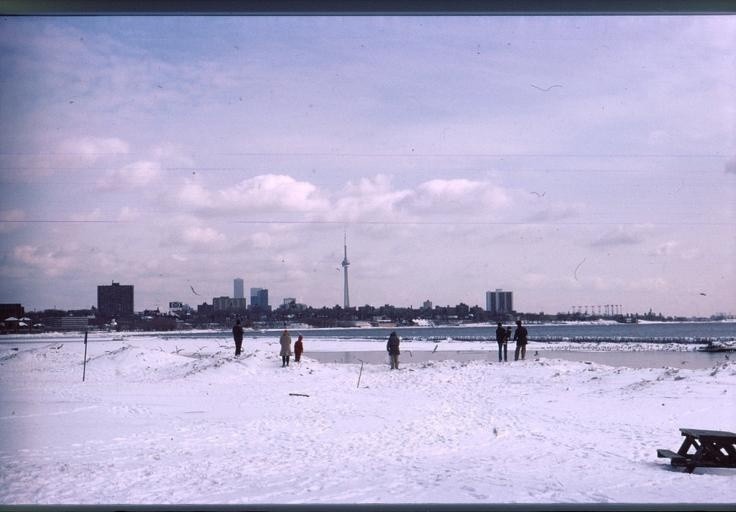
[{"xmin": 670, "ymin": 428, "xmax": 736, "ymax": 474}]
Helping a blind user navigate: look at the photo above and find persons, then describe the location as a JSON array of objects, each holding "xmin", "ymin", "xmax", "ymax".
[
  {"xmin": 294, "ymin": 335, "xmax": 303, "ymax": 361},
  {"xmin": 233, "ymin": 321, "xmax": 242, "ymax": 356},
  {"xmin": 496, "ymin": 320, "xmax": 527, "ymax": 362},
  {"xmin": 387, "ymin": 331, "xmax": 400, "ymax": 369},
  {"xmin": 280, "ymin": 330, "xmax": 291, "ymax": 367}
]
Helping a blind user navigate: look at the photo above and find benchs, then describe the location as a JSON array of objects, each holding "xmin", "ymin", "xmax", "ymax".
[{"xmin": 658, "ymin": 448, "xmax": 690, "ymax": 467}]
